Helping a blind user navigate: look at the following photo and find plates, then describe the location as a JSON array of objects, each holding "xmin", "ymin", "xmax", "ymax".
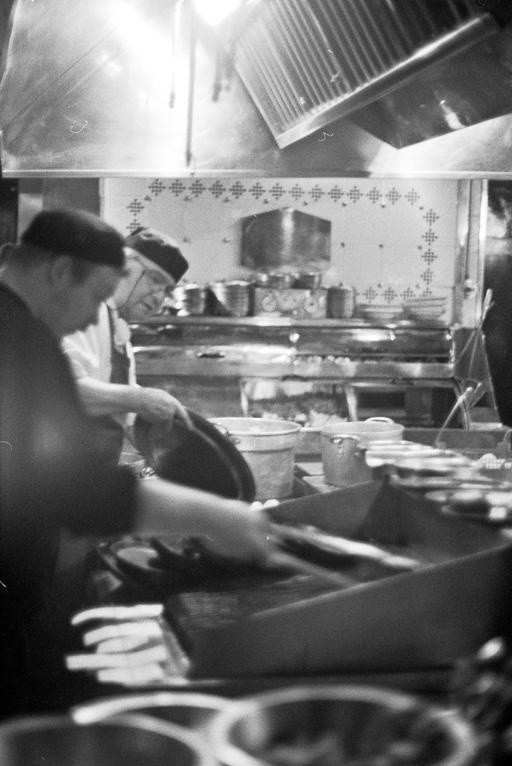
[
  {"xmin": 134, "ymin": 405, "xmax": 253, "ymax": 505},
  {"xmin": 359, "ymin": 296, "xmax": 449, "ymax": 323}
]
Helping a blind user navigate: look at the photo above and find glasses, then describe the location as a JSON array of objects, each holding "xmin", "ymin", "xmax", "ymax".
[{"xmin": 127, "ymin": 252, "xmax": 175, "ymax": 298}]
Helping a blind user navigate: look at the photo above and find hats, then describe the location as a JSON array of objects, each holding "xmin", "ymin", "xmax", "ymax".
[
  {"xmin": 18, "ymin": 205, "xmax": 127, "ymax": 271},
  {"xmin": 120, "ymin": 223, "xmax": 191, "ymax": 284}
]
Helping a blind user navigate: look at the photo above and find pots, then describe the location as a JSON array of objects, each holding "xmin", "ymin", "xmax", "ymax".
[
  {"xmin": 322, "ymin": 414, "xmax": 512, "ymax": 522},
  {"xmin": 199, "ymin": 415, "xmax": 303, "ymax": 504},
  {"xmin": 328, "ymin": 282, "xmax": 359, "ymax": 318}
]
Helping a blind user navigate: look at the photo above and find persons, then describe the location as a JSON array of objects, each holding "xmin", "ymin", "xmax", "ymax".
[
  {"xmin": 0, "ymin": 206, "xmax": 289, "ymax": 733},
  {"xmin": 45, "ymin": 220, "xmax": 196, "ymax": 457}
]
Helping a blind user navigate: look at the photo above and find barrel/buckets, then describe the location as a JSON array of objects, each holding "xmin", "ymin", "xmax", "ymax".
[
  {"xmin": 318, "ymin": 416, "xmax": 404, "ymax": 489},
  {"xmin": 208, "ymin": 418, "xmax": 301, "ymax": 500}
]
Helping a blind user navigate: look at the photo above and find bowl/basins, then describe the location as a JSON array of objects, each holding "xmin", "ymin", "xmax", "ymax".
[{"xmin": 171, "ymin": 270, "xmax": 322, "ymax": 318}]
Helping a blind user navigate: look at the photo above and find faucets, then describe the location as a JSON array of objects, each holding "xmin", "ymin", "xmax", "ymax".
[{"xmin": 432, "ymin": 386, "xmax": 473, "ymax": 446}]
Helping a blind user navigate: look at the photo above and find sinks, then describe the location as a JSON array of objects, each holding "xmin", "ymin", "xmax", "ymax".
[{"xmin": 402, "ymin": 427, "xmax": 512, "ymax": 450}]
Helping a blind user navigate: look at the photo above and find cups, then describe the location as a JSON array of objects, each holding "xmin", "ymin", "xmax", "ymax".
[
  {"xmin": 212, "ymin": 685, "xmax": 476, "ymax": 766},
  {"xmin": 0, "ymin": 713, "xmax": 215, "ymax": 766},
  {"xmin": 78, "ymin": 689, "xmax": 235, "ymax": 730}
]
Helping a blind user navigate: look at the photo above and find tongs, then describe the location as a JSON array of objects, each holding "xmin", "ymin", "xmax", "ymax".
[{"xmin": 250, "ymin": 519, "xmax": 425, "ymax": 594}]
